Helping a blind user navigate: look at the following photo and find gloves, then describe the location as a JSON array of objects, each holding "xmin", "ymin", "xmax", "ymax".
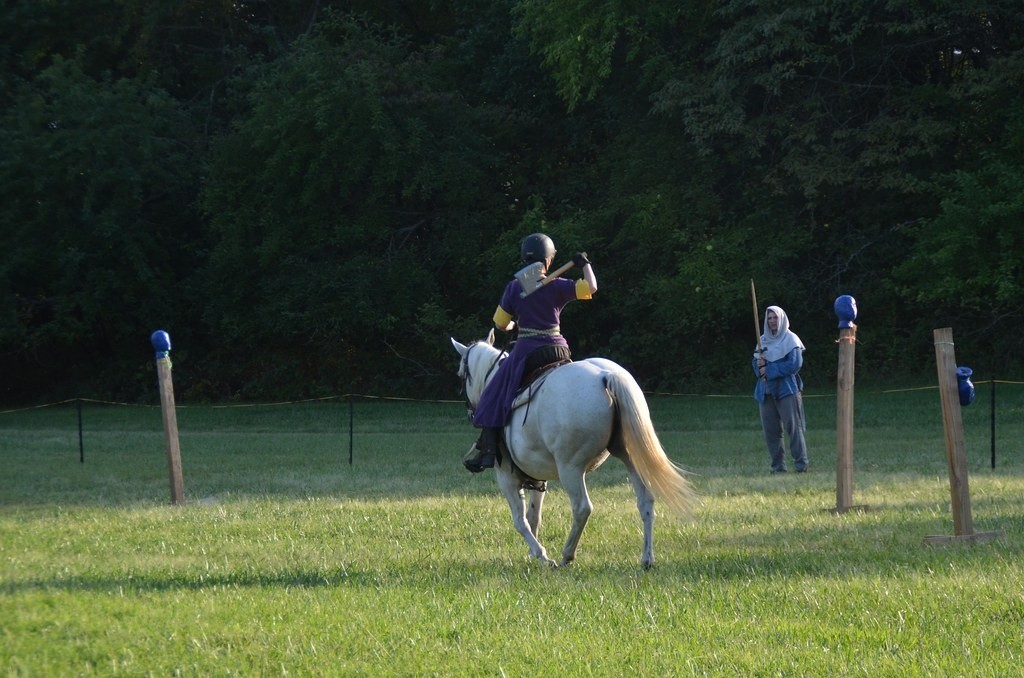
[
  {"xmin": 570, "ymin": 252, "xmax": 592, "ymax": 268},
  {"xmin": 509, "ymin": 319, "xmax": 518, "ymax": 334}
]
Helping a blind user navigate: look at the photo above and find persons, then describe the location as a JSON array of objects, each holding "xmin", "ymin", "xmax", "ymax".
[
  {"xmin": 465, "ymin": 234, "xmax": 597, "ymax": 474},
  {"xmin": 751, "ymin": 306, "xmax": 809, "ymax": 474}
]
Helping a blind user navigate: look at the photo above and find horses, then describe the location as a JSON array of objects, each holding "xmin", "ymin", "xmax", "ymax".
[{"xmin": 450, "ymin": 328, "xmax": 703, "ymax": 572}]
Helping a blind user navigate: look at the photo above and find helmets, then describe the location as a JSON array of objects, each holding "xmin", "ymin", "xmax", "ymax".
[{"xmin": 520, "ymin": 233, "xmax": 557, "ymax": 264}]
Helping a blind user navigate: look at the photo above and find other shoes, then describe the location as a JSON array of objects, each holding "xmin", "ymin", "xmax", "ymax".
[{"xmin": 463, "ymin": 446, "xmax": 494, "ymax": 473}]
